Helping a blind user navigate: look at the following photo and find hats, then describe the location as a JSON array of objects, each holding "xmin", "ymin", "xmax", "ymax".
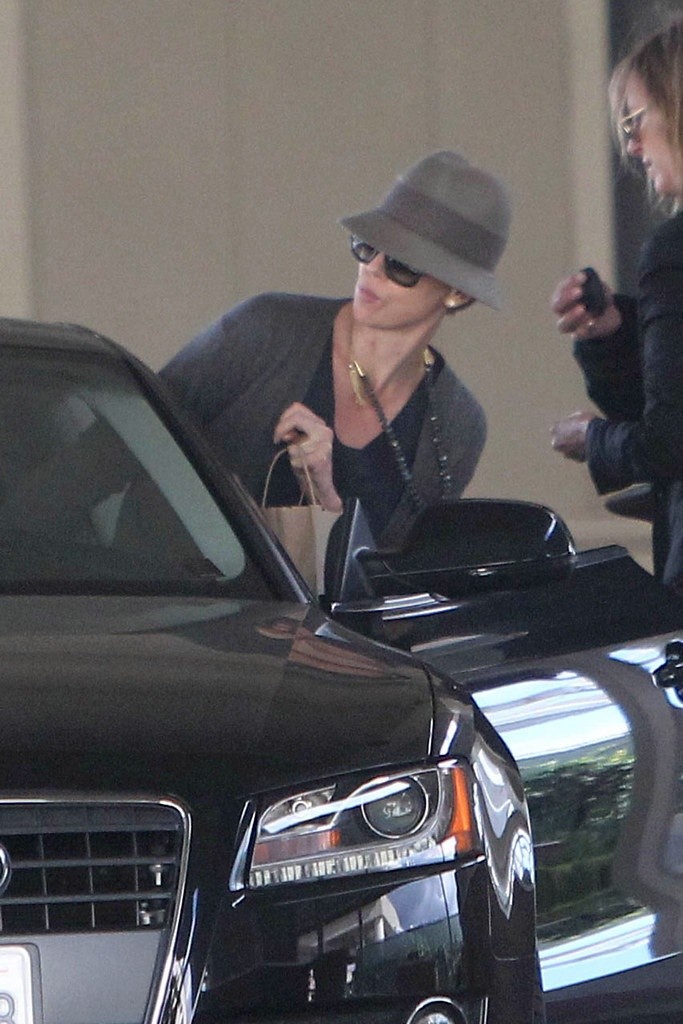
[{"xmin": 342, "ymin": 148, "xmax": 511, "ymax": 311}]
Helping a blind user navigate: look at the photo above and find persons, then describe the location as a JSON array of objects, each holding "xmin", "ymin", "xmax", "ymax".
[
  {"xmin": 18, "ymin": 153, "xmax": 510, "ymax": 595},
  {"xmin": 548, "ymin": 14, "xmax": 683, "ymax": 600}
]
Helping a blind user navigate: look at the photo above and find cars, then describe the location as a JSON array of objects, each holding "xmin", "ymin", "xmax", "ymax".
[{"xmin": 0, "ymin": 317, "xmax": 683, "ymax": 1024}]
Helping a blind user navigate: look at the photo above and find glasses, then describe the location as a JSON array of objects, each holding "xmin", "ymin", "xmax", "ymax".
[
  {"xmin": 619, "ymin": 108, "xmax": 646, "ymax": 137},
  {"xmin": 350, "ymin": 234, "xmax": 423, "ymax": 288}
]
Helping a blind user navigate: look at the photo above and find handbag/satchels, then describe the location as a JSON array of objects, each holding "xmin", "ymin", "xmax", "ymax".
[{"xmin": 256, "ymin": 444, "xmax": 343, "ymax": 597}]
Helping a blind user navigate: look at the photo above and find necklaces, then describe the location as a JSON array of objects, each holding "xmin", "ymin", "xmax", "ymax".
[{"xmin": 351, "ymin": 321, "xmax": 450, "ymax": 512}]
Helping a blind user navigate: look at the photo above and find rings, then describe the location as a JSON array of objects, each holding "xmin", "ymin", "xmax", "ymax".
[{"xmin": 301, "ymin": 440, "xmax": 314, "ymax": 454}]
